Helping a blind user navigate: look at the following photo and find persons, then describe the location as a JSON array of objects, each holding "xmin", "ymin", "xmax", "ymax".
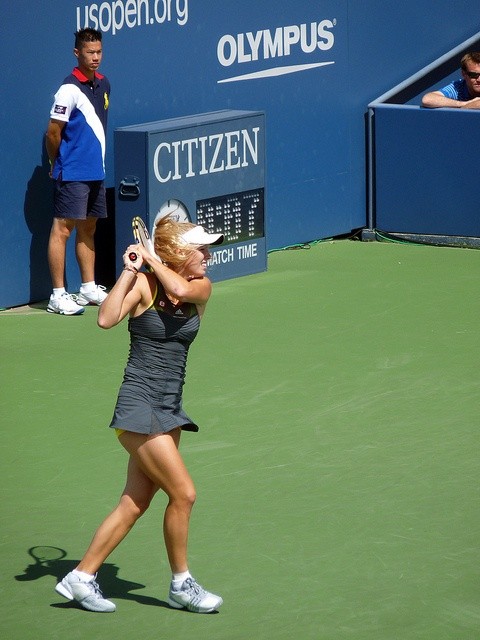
[
  {"xmin": 46, "ymin": 27, "xmax": 111, "ymax": 315},
  {"xmin": 54, "ymin": 221, "xmax": 223, "ymax": 614},
  {"xmin": 421, "ymin": 48, "xmax": 480, "ymax": 109}
]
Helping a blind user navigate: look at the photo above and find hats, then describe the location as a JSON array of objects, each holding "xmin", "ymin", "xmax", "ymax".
[{"xmin": 179, "ymin": 226, "xmax": 225, "ymax": 245}]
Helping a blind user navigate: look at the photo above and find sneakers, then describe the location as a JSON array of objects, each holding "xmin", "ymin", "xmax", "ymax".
[
  {"xmin": 47, "ymin": 294, "xmax": 85, "ymax": 315},
  {"xmin": 55, "ymin": 572, "xmax": 116, "ymax": 612},
  {"xmin": 169, "ymin": 578, "xmax": 223, "ymax": 613},
  {"xmin": 77, "ymin": 285, "xmax": 108, "ymax": 306}
]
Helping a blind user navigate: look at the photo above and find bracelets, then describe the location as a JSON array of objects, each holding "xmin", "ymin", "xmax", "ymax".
[
  {"xmin": 49, "ymin": 160, "xmax": 54, "ymax": 166},
  {"xmin": 123, "ymin": 263, "xmax": 137, "ymax": 274}
]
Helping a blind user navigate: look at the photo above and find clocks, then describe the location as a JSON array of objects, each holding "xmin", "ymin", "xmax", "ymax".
[{"xmin": 151, "ymin": 197, "xmax": 192, "ymax": 245}]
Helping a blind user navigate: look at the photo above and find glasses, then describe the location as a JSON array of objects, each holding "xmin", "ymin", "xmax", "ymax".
[{"xmin": 468, "ymin": 72, "xmax": 480, "ymax": 79}]
